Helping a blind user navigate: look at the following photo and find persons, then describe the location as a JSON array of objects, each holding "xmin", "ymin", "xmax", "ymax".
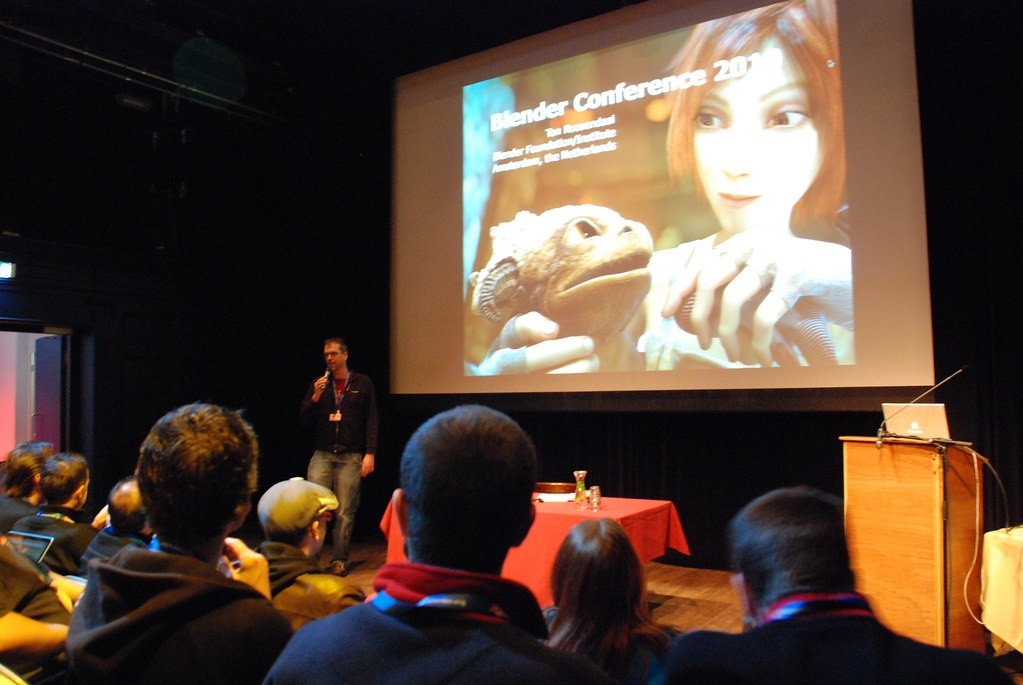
[
  {"xmin": 65, "ymin": 400, "xmax": 294, "ymax": 685},
  {"xmin": 303, "ymin": 335, "xmax": 379, "ymax": 575},
  {"xmin": 262, "ymin": 403, "xmax": 616, "ymax": 685},
  {"xmin": 537, "ymin": 515, "xmax": 678, "ymax": 685},
  {"xmin": 1, "ymin": 402, "xmax": 364, "ymax": 685},
  {"xmin": 662, "ymin": 483, "xmax": 1014, "ymax": 685}
]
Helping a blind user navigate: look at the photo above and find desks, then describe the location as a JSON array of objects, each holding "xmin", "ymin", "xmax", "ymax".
[
  {"xmin": 379, "ymin": 488, "xmax": 692, "ymax": 610},
  {"xmin": 980, "ymin": 523, "xmax": 1023, "ymax": 655}
]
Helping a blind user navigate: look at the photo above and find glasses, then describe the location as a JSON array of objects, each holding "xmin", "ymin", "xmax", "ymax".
[{"xmin": 321, "ymin": 351, "xmax": 344, "ymax": 359}]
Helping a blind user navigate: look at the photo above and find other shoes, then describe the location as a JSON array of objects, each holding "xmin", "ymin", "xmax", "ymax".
[{"xmin": 331, "ymin": 561, "xmax": 346, "ymax": 577}]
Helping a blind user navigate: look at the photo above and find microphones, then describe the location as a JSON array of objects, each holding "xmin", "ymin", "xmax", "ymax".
[
  {"xmin": 876, "ymin": 363, "xmax": 972, "ymax": 448},
  {"xmin": 321, "ymin": 368, "xmax": 332, "ymax": 390}
]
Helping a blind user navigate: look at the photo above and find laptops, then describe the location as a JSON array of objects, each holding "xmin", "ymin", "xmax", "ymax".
[
  {"xmin": 880, "ymin": 402, "xmax": 973, "ymax": 447},
  {"xmin": 5, "ymin": 530, "xmax": 54, "ymax": 565}
]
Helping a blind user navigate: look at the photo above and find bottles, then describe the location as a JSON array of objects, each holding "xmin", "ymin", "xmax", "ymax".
[
  {"xmin": 590, "ymin": 485, "xmax": 601, "ymax": 512},
  {"xmin": 573, "ymin": 471, "xmax": 588, "ymax": 511}
]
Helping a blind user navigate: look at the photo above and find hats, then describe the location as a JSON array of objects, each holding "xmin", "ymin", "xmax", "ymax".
[{"xmin": 256, "ymin": 479, "xmax": 338, "ymax": 537}]
